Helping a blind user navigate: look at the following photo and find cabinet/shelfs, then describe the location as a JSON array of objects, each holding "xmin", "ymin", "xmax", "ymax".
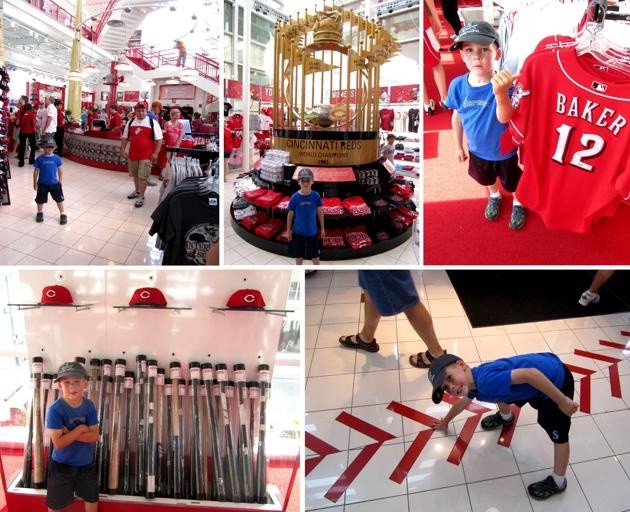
[
  {"xmin": 384, "ymin": 139, "xmax": 420, "ymax": 178},
  {"xmin": 230, "ymin": 157, "xmax": 417, "ymax": 261}
]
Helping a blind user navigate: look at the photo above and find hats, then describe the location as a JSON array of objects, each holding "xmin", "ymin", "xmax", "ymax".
[
  {"xmin": 54, "ymin": 362, "xmax": 86, "ymax": 383},
  {"xmin": 152, "ymin": 102, "xmax": 165, "ymax": 110},
  {"xmin": 428, "ymin": 354, "xmax": 460, "ymax": 404},
  {"xmin": 224, "ymin": 288, "xmax": 268, "ymax": 309},
  {"xmin": 44, "ymin": 138, "xmax": 57, "ymax": 148},
  {"xmin": 41, "ymin": 285, "xmax": 75, "ymax": 307},
  {"xmin": 136, "ymin": 101, "xmax": 147, "ymax": 107},
  {"xmin": 298, "ymin": 169, "xmax": 314, "ymax": 179},
  {"xmin": 129, "ymin": 287, "xmax": 167, "ymax": 307},
  {"xmin": 449, "ymin": 21, "xmax": 500, "ymax": 51}
]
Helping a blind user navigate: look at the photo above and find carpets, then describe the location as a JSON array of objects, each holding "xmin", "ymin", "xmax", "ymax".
[
  {"xmin": 446, "ymin": 270, "xmax": 630, "ymax": 329},
  {"xmin": 423, "ymin": 0, "xmax": 482, "ymax": 131},
  {"xmin": 424, "ymin": 132, "xmax": 439, "ymax": 159},
  {"xmin": 423, "ymin": 195, "xmax": 630, "ymax": 265}
]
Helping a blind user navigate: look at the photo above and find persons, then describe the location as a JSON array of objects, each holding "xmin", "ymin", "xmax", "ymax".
[
  {"xmin": 8, "ymin": 95, "xmax": 218, "ymax": 224},
  {"xmin": 340, "ymin": 271, "xmax": 446, "ymax": 368},
  {"xmin": 444, "ymin": 21, "xmax": 527, "ymax": 228},
  {"xmin": 383, "ymin": 134, "xmax": 397, "ymax": 166},
  {"xmin": 428, "ymin": 352, "xmax": 581, "ymax": 501},
  {"xmin": 579, "ymin": 270, "xmax": 614, "ymax": 308},
  {"xmin": 173, "ymin": 39, "xmax": 187, "ymax": 66},
  {"xmin": 45, "ymin": 363, "xmax": 101, "ymax": 508},
  {"xmin": 224, "ymin": 124, "xmax": 233, "ymax": 182},
  {"xmin": 287, "ymin": 169, "xmax": 326, "ymax": 265}
]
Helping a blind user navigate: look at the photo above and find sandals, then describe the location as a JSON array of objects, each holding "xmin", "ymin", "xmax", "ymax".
[
  {"xmin": 339, "ymin": 334, "xmax": 379, "ymax": 352},
  {"xmin": 409, "ymin": 350, "xmax": 447, "ymax": 368}
]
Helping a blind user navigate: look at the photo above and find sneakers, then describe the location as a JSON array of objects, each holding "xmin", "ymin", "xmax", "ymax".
[
  {"xmin": 481, "ymin": 411, "xmax": 515, "ymax": 429},
  {"xmin": 440, "ymin": 100, "xmax": 449, "ymax": 110},
  {"xmin": 485, "ymin": 195, "xmax": 500, "ymax": 220},
  {"xmin": 135, "ymin": 197, "xmax": 144, "ymax": 208},
  {"xmin": 128, "ymin": 192, "xmax": 139, "ymax": 199},
  {"xmin": 60, "ymin": 214, "xmax": 67, "ymax": 225},
  {"xmin": 37, "ymin": 212, "xmax": 43, "ymax": 222},
  {"xmin": 579, "ymin": 291, "xmax": 600, "ymax": 307},
  {"xmin": 528, "ymin": 475, "xmax": 567, "ymax": 501},
  {"xmin": 424, "ymin": 99, "xmax": 435, "ymax": 112},
  {"xmin": 509, "ymin": 205, "xmax": 525, "ymax": 229}
]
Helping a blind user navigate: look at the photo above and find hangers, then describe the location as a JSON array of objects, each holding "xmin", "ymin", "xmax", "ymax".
[
  {"xmin": 172, "ymin": 147, "xmax": 199, "ymax": 167},
  {"xmin": 190, "ymin": 161, "xmax": 219, "ymax": 200},
  {"xmin": 545, "ymin": 0, "xmax": 626, "ymax": 74}
]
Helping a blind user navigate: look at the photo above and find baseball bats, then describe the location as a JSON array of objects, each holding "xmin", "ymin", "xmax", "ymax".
[{"xmin": 15, "ymin": 354, "xmax": 272, "ymax": 505}]
